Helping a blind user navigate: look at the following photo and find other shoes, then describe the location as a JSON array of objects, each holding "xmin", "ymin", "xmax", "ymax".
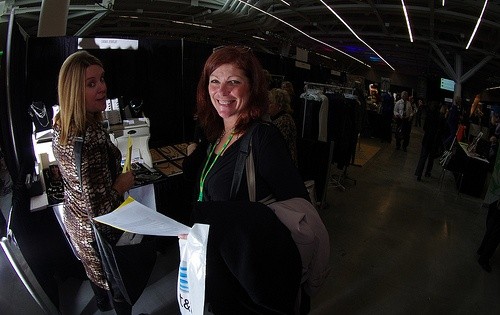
[
  {"xmin": 403, "ymin": 144, "xmax": 406, "ymax": 152},
  {"xmin": 381, "ymin": 138, "xmax": 386, "ymax": 144},
  {"xmin": 396, "ymin": 143, "xmax": 401, "ymax": 150},
  {"xmin": 417, "ymin": 176, "xmax": 421, "ymax": 183},
  {"xmin": 425, "ymin": 172, "xmax": 432, "ymax": 176},
  {"xmin": 478, "ymin": 257, "xmax": 491, "ymax": 273},
  {"xmin": 387, "ymin": 137, "xmax": 391, "ymax": 144},
  {"xmin": 478, "ymin": 249, "xmax": 492, "ymax": 258}
]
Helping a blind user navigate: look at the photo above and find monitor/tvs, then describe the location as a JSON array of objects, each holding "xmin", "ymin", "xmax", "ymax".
[{"xmin": 440, "ymin": 78, "xmax": 455, "ymax": 92}]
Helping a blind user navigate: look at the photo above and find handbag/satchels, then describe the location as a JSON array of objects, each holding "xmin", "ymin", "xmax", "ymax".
[
  {"xmin": 431, "ymin": 140, "xmax": 445, "ymax": 157},
  {"xmin": 94, "ymin": 228, "xmax": 164, "ymax": 306}
]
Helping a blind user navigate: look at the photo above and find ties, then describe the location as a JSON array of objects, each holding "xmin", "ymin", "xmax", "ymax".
[{"xmin": 404, "ymin": 101, "xmax": 406, "ymax": 118}]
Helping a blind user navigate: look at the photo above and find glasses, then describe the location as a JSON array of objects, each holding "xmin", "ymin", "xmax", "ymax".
[{"xmin": 213, "ymin": 45, "xmax": 253, "ymax": 55}]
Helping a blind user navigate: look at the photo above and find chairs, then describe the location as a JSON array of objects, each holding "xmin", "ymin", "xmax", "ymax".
[{"xmin": 439, "ymin": 151, "xmax": 464, "ymax": 192}]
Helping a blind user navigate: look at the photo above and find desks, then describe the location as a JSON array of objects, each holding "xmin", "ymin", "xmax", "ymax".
[
  {"xmin": 29, "ymin": 140, "xmax": 226, "ymax": 240},
  {"xmin": 455, "ymin": 141, "xmax": 490, "ymax": 198}
]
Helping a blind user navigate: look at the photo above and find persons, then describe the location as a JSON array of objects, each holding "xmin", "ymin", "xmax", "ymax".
[
  {"xmin": 382, "ymin": 89, "xmax": 394, "ymax": 144},
  {"xmin": 393, "ymin": 91, "xmax": 413, "ymax": 152},
  {"xmin": 131, "ymin": 46, "xmax": 313, "ymax": 315},
  {"xmin": 52, "ymin": 51, "xmax": 136, "ymax": 315},
  {"xmin": 477, "ymin": 135, "xmax": 500, "ymax": 276},
  {"xmin": 392, "ymin": 88, "xmax": 500, "ymax": 139},
  {"xmin": 415, "ymin": 118, "xmax": 449, "ymax": 181},
  {"xmin": 481, "ymin": 128, "xmax": 497, "ymax": 140}
]
